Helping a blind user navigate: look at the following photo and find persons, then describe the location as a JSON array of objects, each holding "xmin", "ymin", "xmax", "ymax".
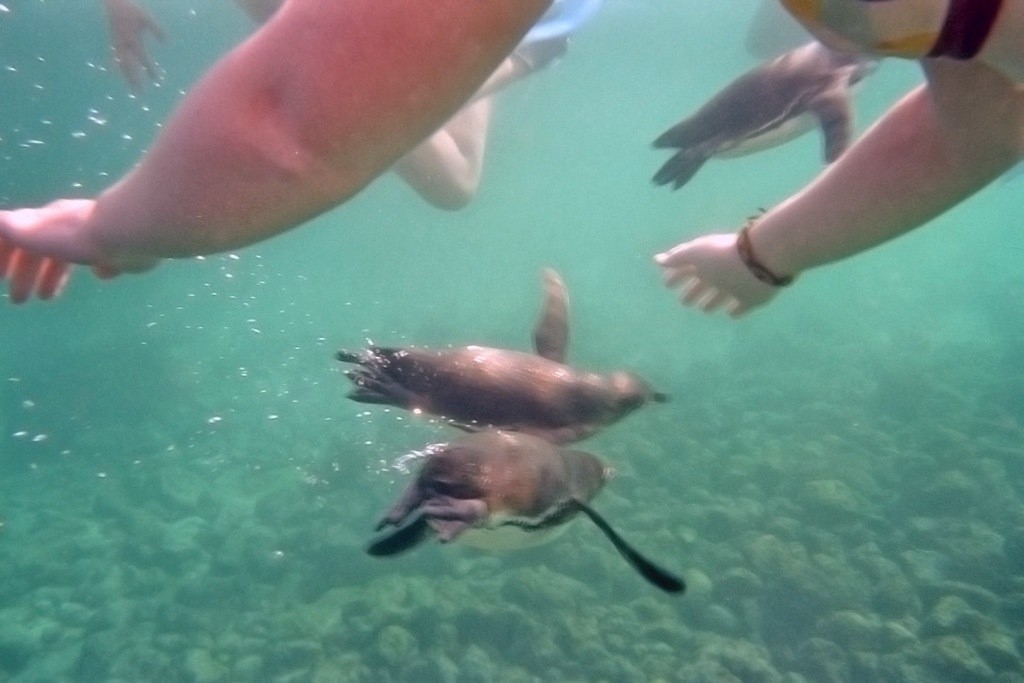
[
  {"xmin": 0, "ymin": 0, "xmax": 1024, "ymax": 321},
  {"xmin": 100, "ymin": 1, "xmax": 596, "ymax": 212}
]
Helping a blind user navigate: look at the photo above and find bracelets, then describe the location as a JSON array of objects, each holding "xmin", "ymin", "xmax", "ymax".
[{"xmin": 737, "ymin": 214, "xmax": 794, "ymax": 287}]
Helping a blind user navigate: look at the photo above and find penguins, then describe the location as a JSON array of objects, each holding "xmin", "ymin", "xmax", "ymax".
[
  {"xmin": 649, "ymin": 38, "xmax": 881, "ymax": 192},
  {"xmin": 336, "ymin": 265, "xmax": 685, "ymax": 595}
]
